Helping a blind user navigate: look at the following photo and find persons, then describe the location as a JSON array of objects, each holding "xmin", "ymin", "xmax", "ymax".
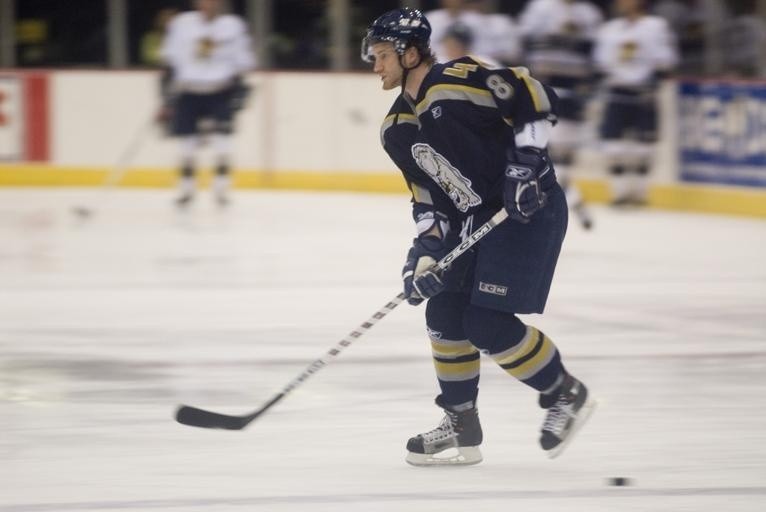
[{"xmin": 356, "ymin": 3, "xmax": 591, "ymax": 458}]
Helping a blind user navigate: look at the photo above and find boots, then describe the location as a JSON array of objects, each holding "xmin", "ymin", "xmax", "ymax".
[
  {"xmin": 538, "ymin": 372, "xmax": 587, "ymax": 451},
  {"xmin": 406, "ymin": 392, "xmax": 483, "ymax": 453},
  {"xmin": 175, "ymin": 172, "xmax": 233, "ymax": 204}
]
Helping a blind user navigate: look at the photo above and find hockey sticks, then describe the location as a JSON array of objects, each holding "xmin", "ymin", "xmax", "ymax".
[{"xmin": 175, "ymin": 208, "xmax": 509, "ymax": 431}]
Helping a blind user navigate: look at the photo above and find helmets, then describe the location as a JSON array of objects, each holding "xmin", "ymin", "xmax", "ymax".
[{"xmin": 361, "ymin": 7, "xmax": 431, "ymax": 64}]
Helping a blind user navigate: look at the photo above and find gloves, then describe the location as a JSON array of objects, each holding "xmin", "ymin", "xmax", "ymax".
[
  {"xmin": 403, "ymin": 235, "xmax": 449, "ymax": 305},
  {"xmin": 504, "ymin": 159, "xmax": 547, "ymax": 223}
]
